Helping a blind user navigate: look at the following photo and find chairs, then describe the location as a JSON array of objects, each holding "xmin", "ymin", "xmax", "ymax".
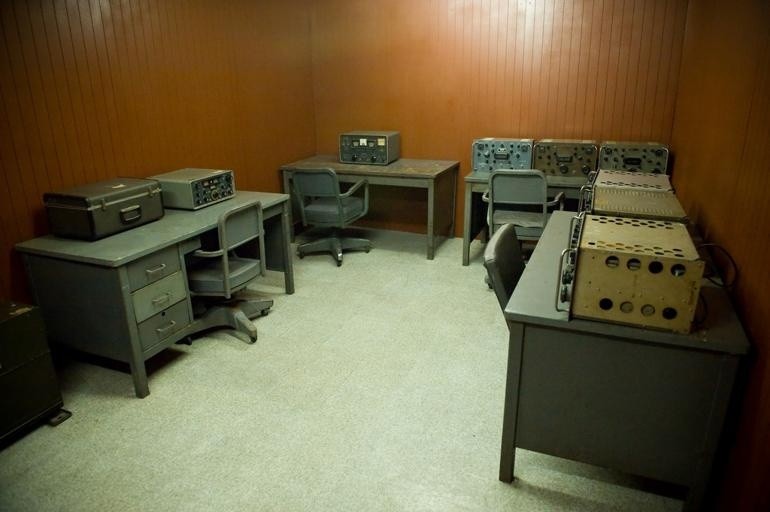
[
  {"xmin": 291, "ymin": 167, "xmax": 374, "ymax": 266},
  {"xmin": 482, "ymin": 222, "xmax": 526, "ymax": 312},
  {"xmin": 183, "ymin": 201, "xmax": 274, "ymax": 346},
  {"xmin": 481, "ymin": 169, "xmax": 564, "ymax": 290}
]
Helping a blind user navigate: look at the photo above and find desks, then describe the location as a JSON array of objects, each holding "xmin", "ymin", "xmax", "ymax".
[
  {"xmin": 498, "ymin": 211, "xmax": 747, "ymax": 508},
  {"xmin": 18, "ymin": 189, "xmax": 295, "ymax": 398},
  {"xmin": 280, "ymin": 156, "xmax": 460, "ymax": 260},
  {"xmin": 463, "ymin": 167, "xmax": 592, "ymax": 266}
]
